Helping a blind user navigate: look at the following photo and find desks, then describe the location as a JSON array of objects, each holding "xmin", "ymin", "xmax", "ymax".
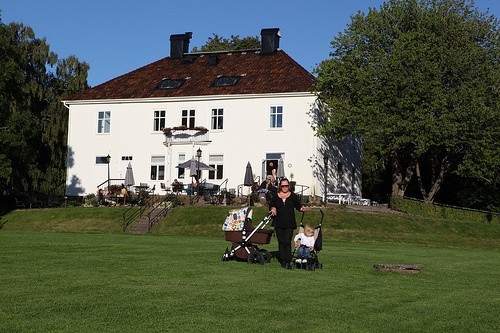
[
  {"xmin": 170, "ymin": 184, "xmax": 184, "ymax": 195},
  {"xmin": 134, "ymin": 186, "xmax": 149, "ymax": 193},
  {"xmin": 103, "ymin": 195, "xmax": 124, "ymax": 205}
]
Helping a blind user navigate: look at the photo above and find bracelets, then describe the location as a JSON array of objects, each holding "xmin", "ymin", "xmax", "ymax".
[{"xmin": 301, "ymin": 208, "xmax": 302, "ymax": 211}]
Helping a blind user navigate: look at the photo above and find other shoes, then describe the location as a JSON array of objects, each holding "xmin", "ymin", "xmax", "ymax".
[
  {"xmin": 301, "ymin": 259, "xmax": 308, "ymax": 263},
  {"xmin": 281, "ymin": 262, "xmax": 292, "ymax": 269},
  {"xmin": 295, "ymin": 258, "xmax": 302, "ymax": 266}
]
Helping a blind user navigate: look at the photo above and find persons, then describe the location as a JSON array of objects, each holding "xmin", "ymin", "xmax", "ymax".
[
  {"xmin": 193, "ymin": 176, "xmax": 198, "ymax": 195},
  {"xmin": 99, "ymin": 189, "xmax": 105, "ymax": 208},
  {"xmin": 269, "ymin": 178, "xmax": 308, "ymax": 268},
  {"xmin": 172, "ymin": 179, "xmax": 182, "ymax": 196},
  {"xmin": 260, "ymin": 169, "xmax": 278, "ymax": 202},
  {"xmin": 294, "ymin": 223, "xmax": 315, "ymax": 265},
  {"xmin": 121, "ymin": 183, "xmax": 127, "ymax": 206}
]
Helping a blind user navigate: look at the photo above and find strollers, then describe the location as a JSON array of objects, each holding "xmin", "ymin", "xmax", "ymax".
[
  {"xmin": 221, "ymin": 207, "xmax": 275, "ymax": 264},
  {"xmin": 286, "ymin": 205, "xmax": 324, "ymax": 271}
]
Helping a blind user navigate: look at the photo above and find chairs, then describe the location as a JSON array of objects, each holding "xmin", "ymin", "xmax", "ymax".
[
  {"xmin": 160, "ymin": 183, "xmax": 171, "ymax": 194},
  {"xmin": 140, "ymin": 182, "xmax": 156, "ymax": 196},
  {"xmin": 15, "ymin": 196, "xmax": 42, "ymax": 209},
  {"xmin": 177, "ymin": 182, "xmax": 185, "ymax": 195}
]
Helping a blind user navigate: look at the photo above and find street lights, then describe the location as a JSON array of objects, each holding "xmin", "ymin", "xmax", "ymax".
[
  {"xmin": 322, "ymin": 153, "xmax": 329, "ymax": 206},
  {"xmin": 197, "ymin": 147, "xmax": 202, "ymax": 200},
  {"xmin": 106, "ymin": 153, "xmax": 111, "ymax": 195}
]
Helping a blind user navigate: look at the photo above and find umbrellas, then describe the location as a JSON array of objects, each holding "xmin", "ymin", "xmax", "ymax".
[
  {"xmin": 175, "ymin": 156, "xmax": 217, "ymax": 183},
  {"xmin": 124, "ymin": 163, "xmax": 135, "ymax": 205},
  {"xmin": 190, "ymin": 156, "xmax": 196, "ymax": 177},
  {"xmin": 244, "ymin": 161, "xmax": 254, "ymax": 206}
]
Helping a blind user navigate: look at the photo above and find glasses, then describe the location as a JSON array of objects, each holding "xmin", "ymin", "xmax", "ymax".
[{"xmin": 281, "ymin": 185, "xmax": 290, "ymax": 188}]
